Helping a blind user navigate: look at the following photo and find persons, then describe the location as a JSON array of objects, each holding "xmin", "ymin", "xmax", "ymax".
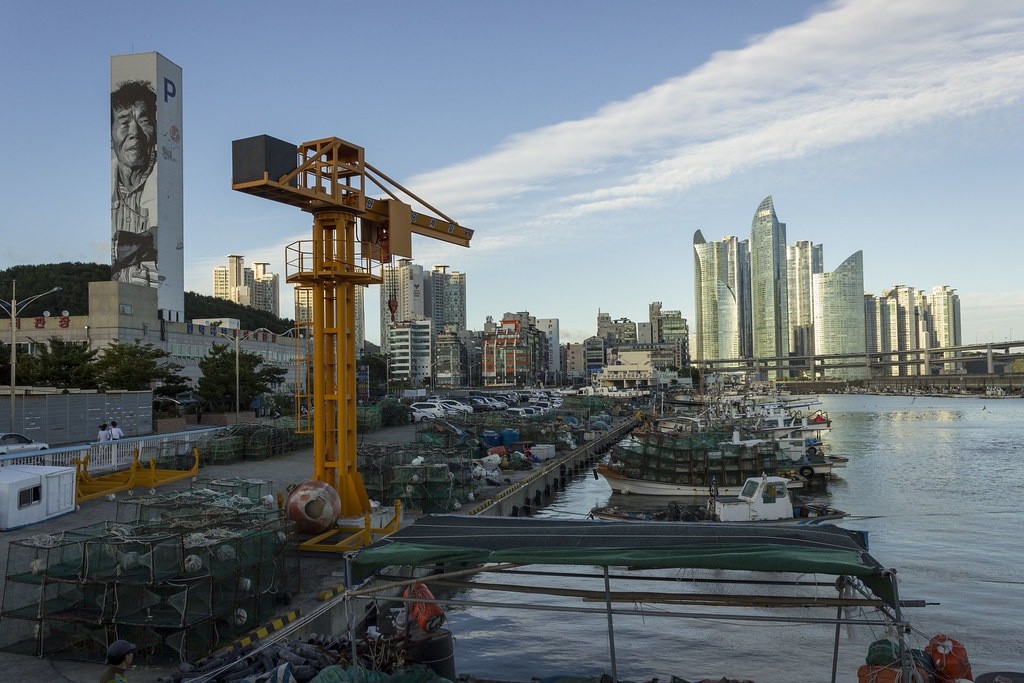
[
  {"xmin": 109, "ymin": 421, "xmax": 124, "ymax": 441},
  {"xmin": 196, "ymin": 404, "xmax": 202, "ymax": 424},
  {"xmin": 110, "ymin": 80, "xmax": 158, "ymax": 288},
  {"xmin": 97, "ymin": 424, "xmax": 110, "ymax": 442}
]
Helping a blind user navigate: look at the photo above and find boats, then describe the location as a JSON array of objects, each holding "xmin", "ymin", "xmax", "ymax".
[
  {"xmin": 855, "ymin": 371, "xmax": 1023, "ymax": 399},
  {"xmin": 589, "ymin": 370, "xmax": 856, "ymax": 529}
]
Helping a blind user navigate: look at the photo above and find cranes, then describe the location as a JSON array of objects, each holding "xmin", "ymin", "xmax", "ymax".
[{"xmin": 230, "ymin": 137, "xmax": 477, "ymax": 523}]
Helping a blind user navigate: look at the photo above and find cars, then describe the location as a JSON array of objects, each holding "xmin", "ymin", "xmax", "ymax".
[
  {"xmin": 405, "ymin": 384, "xmax": 566, "ymax": 424},
  {"xmin": 0, "ymin": 433, "xmax": 50, "ymax": 457}
]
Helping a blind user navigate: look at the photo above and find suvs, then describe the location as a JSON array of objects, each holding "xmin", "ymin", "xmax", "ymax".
[{"xmin": 153, "ymin": 397, "xmax": 202, "ymax": 422}]
